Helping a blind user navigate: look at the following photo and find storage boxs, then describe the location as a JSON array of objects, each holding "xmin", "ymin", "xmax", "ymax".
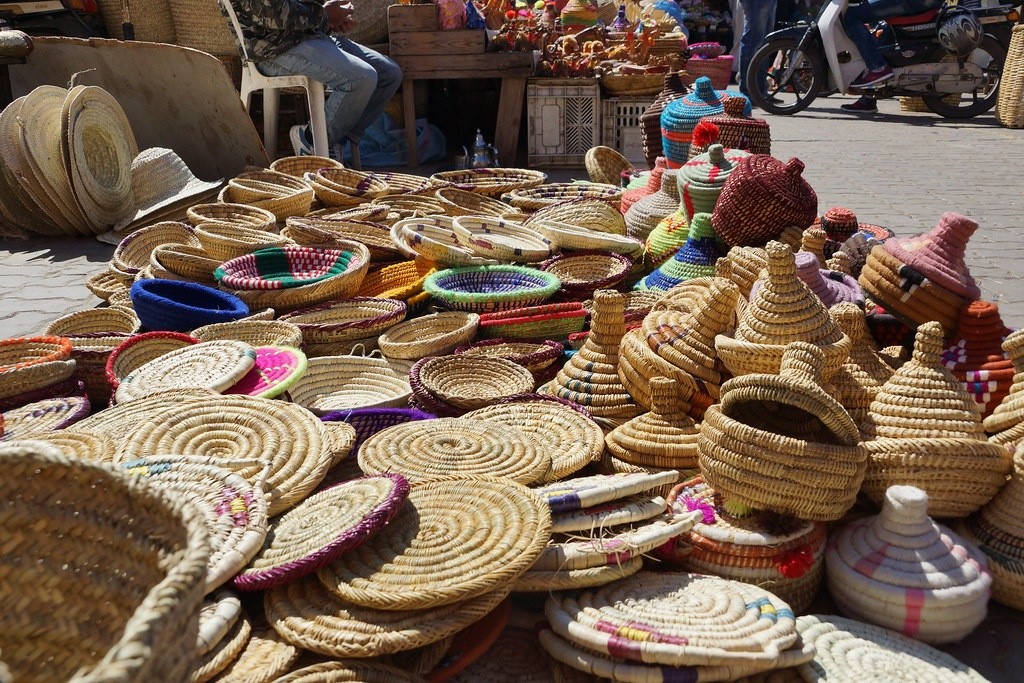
[
  {"xmin": 601, "ymin": 99, "xmax": 655, "ymax": 163},
  {"xmin": 525, "ymin": 76, "xmax": 600, "ymax": 171}
]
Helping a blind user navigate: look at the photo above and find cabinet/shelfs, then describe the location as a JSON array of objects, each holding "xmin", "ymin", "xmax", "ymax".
[{"xmin": 386, "ymin": 3, "xmax": 534, "ymax": 169}]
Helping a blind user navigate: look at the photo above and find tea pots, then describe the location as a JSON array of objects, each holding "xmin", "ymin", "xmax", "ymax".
[{"xmin": 462, "ymin": 128, "xmax": 500, "ymax": 169}]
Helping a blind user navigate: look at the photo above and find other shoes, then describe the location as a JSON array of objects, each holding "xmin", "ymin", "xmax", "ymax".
[{"xmin": 751, "ymin": 94, "xmax": 784, "ymax": 109}]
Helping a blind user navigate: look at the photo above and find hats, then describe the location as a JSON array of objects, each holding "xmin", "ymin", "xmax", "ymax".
[{"xmin": 0, "ymin": 69, "xmax": 225, "ymax": 244}]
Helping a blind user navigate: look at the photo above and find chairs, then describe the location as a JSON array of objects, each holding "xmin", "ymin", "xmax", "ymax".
[{"xmin": 222, "ymin": 0, "xmax": 362, "ymax": 171}]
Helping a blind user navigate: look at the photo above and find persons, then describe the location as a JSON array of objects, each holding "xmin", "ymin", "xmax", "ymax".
[
  {"xmin": 739, "ymin": 0, "xmax": 785, "ymax": 109},
  {"xmin": 217, "ymin": 0, "xmax": 402, "ymax": 163},
  {"xmin": 839, "ymin": 0, "xmax": 945, "ymax": 113}
]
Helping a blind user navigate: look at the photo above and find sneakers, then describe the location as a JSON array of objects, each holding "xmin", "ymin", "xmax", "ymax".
[
  {"xmin": 329, "ymin": 143, "xmax": 350, "ymax": 169},
  {"xmin": 840, "ymin": 95, "xmax": 879, "ymax": 113},
  {"xmin": 849, "ymin": 65, "xmax": 894, "ymax": 87},
  {"xmin": 290, "ymin": 123, "xmax": 314, "ymax": 156}
]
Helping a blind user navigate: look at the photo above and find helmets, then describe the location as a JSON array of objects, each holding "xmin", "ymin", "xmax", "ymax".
[{"xmin": 936, "ymin": 6, "xmax": 984, "ymax": 58}]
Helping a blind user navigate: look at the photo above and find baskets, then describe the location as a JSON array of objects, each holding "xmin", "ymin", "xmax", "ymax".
[
  {"xmin": 3, "ymin": 145, "xmax": 638, "ymax": 397},
  {"xmin": 561, "ymin": 0, "xmax": 734, "ymax": 96}
]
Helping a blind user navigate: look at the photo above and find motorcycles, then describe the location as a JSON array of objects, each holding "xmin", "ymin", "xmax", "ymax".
[{"xmin": 747, "ymin": 0, "xmax": 1005, "ymax": 119}]
